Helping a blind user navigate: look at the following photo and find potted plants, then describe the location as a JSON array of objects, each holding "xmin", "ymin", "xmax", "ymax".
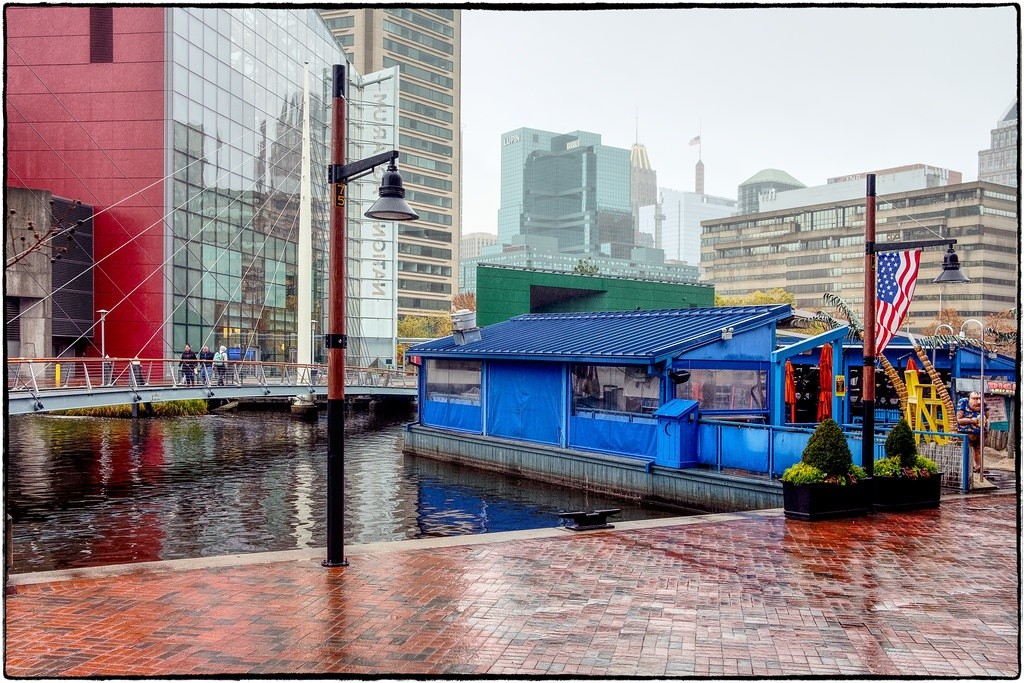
[
  {"xmin": 776, "ymin": 418, "xmax": 872, "ymax": 522},
  {"xmin": 872, "ymin": 419, "xmax": 945, "ymax": 512}
]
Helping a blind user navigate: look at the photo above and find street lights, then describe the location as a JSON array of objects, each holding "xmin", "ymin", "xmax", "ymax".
[
  {"xmin": 929, "ymin": 324, "xmax": 954, "ymax": 386},
  {"xmin": 311, "ymin": 320, "xmax": 319, "ymax": 370},
  {"xmin": 859, "ymin": 168, "xmax": 975, "ymax": 475},
  {"xmin": 957, "ymin": 320, "xmax": 986, "ymax": 484},
  {"xmin": 98, "ymin": 309, "xmax": 109, "ymax": 387},
  {"xmin": 323, "ymin": 66, "xmax": 420, "ymax": 567}
]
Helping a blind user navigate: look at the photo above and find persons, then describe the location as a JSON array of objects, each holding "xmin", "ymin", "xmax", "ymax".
[
  {"xmin": 200, "ymin": 345, "xmax": 214, "ymax": 386},
  {"xmin": 177, "ymin": 343, "xmax": 198, "ymax": 387},
  {"xmin": 212, "ymin": 345, "xmax": 228, "ymax": 386},
  {"xmin": 955, "ymin": 391, "xmax": 990, "ymax": 472}
]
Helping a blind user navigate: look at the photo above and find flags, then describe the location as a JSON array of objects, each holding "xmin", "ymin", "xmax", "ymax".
[
  {"xmin": 689, "ymin": 135, "xmax": 700, "ymax": 146},
  {"xmin": 875, "ymin": 248, "xmax": 921, "ymax": 358}
]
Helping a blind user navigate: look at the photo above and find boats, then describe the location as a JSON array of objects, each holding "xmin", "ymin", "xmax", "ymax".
[{"xmin": 398, "ymin": 306, "xmax": 1014, "ymax": 514}]
